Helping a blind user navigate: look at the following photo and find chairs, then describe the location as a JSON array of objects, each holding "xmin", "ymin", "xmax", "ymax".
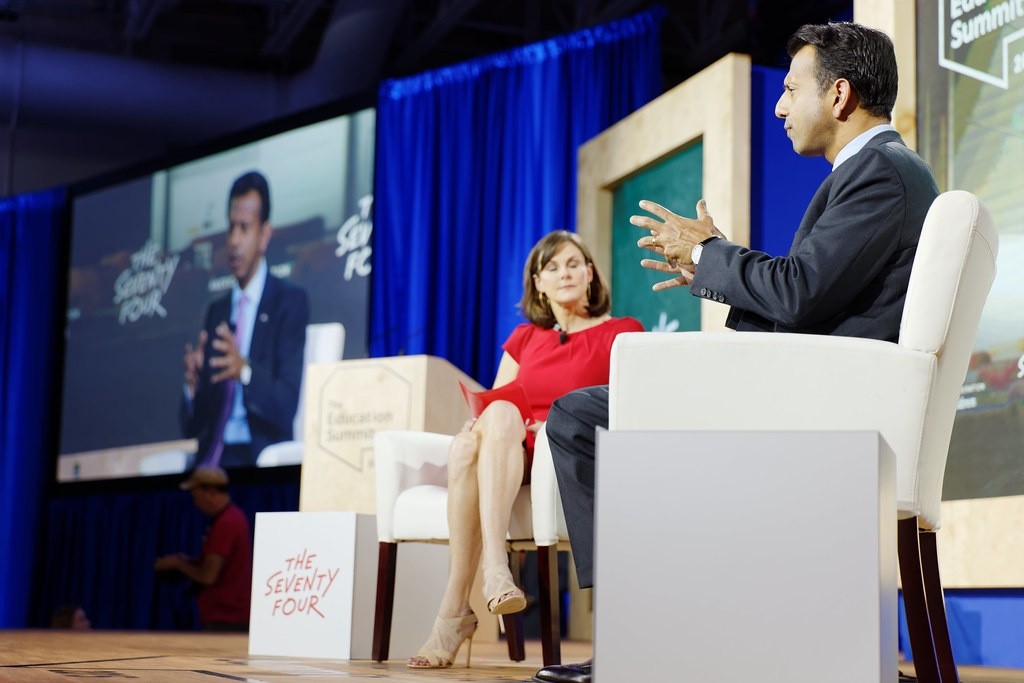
[
  {"xmin": 371, "ymin": 417, "xmax": 576, "ymax": 671},
  {"xmin": 608, "ymin": 189, "xmax": 1001, "ymax": 683}
]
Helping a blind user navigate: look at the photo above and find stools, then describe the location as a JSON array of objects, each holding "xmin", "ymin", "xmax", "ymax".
[{"xmin": 594, "ymin": 427, "xmax": 900, "ymax": 683}]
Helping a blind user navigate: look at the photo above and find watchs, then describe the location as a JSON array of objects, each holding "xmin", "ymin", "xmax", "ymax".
[{"xmin": 691, "ymin": 237, "xmax": 718, "ymax": 266}]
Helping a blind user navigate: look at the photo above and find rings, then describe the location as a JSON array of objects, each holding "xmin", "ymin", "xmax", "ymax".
[{"xmin": 652, "ymin": 236, "xmax": 656, "ymax": 246}]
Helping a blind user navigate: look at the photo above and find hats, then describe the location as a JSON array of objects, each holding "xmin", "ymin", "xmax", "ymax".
[{"xmin": 181, "ymin": 465, "xmax": 228, "ymax": 491}]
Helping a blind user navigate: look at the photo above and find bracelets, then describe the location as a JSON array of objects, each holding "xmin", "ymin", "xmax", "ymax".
[{"xmin": 240, "ymin": 364, "xmax": 252, "ymax": 385}]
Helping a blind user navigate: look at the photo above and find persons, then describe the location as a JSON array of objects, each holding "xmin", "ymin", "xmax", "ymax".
[
  {"xmin": 155, "ymin": 464, "xmax": 252, "ymax": 634},
  {"xmin": 406, "ymin": 230, "xmax": 645, "ymax": 668},
  {"xmin": 537, "ymin": 22, "xmax": 941, "ymax": 683},
  {"xmin": 177, "ymin": 171, "xmax": 310, "ymax": 468},
  {"xmin": 53, "ymin": 606, "xmax": 91, "ymax": 629}
]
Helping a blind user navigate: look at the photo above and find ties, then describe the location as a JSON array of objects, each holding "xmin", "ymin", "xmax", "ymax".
[{"xmin": 209, "ymin": 295, "xmax": 249, "ymax": 467}]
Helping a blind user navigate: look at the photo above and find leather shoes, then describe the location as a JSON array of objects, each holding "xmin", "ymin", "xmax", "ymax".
[{"xmin": 531, "ymin": 655, "xmax": 592, "ymax": 683}]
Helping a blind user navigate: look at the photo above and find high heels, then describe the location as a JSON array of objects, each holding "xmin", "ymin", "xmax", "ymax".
[
  {"xmin": 482, "ymin": 569, "xmax": 527, "ymax": 633},
  {"xmin": 407, "ymin": 609, "xmax": 478, "ymax": 667}
]
